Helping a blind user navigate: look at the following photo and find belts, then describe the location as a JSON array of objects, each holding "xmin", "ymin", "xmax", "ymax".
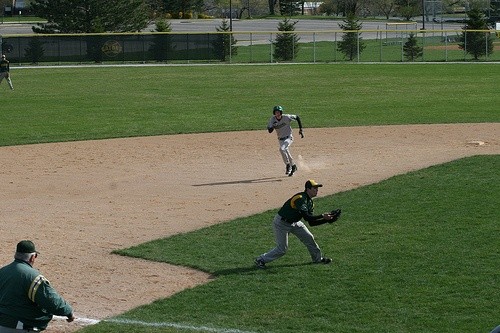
[
  {"xmin": 280, "ymin": 217, "xmax": 296, "ymax": 224},
  {"xmin": 278, "ymin": 136, "xmax": 288, "ymax": 140}
]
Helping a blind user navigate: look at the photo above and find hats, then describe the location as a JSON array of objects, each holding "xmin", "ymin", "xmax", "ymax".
[
  {"xmin": 2, "ymin": 55, "xmax": 6, "ymax": 58},
  {"xmin": 305, "ymin": 180, "xmax": 322, "ymax": 189},
  {"xmin": 17, "ymin": 240, "xmax": 41, "ymax": 254}
]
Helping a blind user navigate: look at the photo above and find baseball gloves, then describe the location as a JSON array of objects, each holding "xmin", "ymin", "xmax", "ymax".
[{"xmin": 328, "ymin": 209, "xmax": 341, "ymax": 223}]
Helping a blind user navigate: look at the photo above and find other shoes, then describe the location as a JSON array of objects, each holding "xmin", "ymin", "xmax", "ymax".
[
  {"xmin": 288, "ymin": 164, "xmax": 297, "ymax": 176},
  {"xmin": 285, "ymin": 163, "xmax": 291, "ymax": 174}
]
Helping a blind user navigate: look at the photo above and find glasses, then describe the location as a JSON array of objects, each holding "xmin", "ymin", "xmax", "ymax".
[{"xmin": 34, "ymin": 253, "xmax": 38, "ymax": 258}]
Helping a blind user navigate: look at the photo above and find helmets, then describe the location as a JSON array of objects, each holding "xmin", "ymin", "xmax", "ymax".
[{"xmin": 272, "ymin": 105, "xmax": 283, "ymax": 115}]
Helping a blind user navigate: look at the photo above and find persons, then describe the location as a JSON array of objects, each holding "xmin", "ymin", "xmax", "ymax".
[
  {"xmin": 267, "ymin": 105, "xmax": 304, "ymax": 177},
  {"xmin": 253, "ymin": 179, "xmax": 342, "ymax": 269},
  {"xmin": 0, "ymin": 54, "xmax": 15, "ymax": 90},
  {"xmin": 0, "ymin": 238, "xmax": 74, "ymax": 333}
]
